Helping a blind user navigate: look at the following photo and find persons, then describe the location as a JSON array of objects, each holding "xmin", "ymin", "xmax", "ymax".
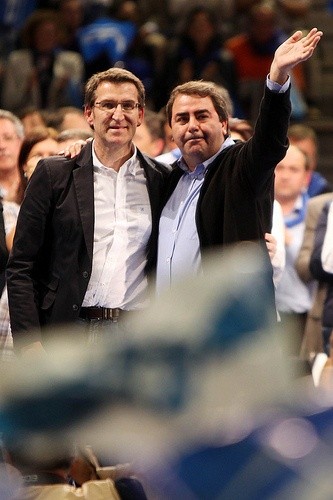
[{"xmin": 0, "ymin": 0, "xmax": 332, "ymax": 499}]
